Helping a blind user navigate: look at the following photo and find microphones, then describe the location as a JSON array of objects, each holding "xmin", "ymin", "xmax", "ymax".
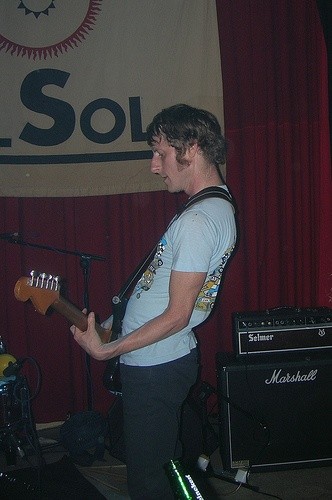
[{"xmin": 0, "ymin": 232, "xmax": 18, "ymax": 239}]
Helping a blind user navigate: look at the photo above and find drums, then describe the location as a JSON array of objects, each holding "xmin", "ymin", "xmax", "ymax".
[{"xmin": 0, "ymin": 375, "xmax": 28, "ymax": 429}]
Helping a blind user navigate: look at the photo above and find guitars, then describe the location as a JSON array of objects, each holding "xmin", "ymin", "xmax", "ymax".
[{"xmin": 14, "ymin": 269, "xmax": 128, "ymax": 397}]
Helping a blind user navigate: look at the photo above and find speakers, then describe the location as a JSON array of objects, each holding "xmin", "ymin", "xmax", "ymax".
[{"xmin": 216, "ymin": 351, "xmax": 332, "ymax": 474}]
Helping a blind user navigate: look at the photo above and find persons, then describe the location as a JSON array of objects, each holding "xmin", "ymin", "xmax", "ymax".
[{"xmin": 69, "ymin": 103, "xmax": 237, "ymax": 500}]
